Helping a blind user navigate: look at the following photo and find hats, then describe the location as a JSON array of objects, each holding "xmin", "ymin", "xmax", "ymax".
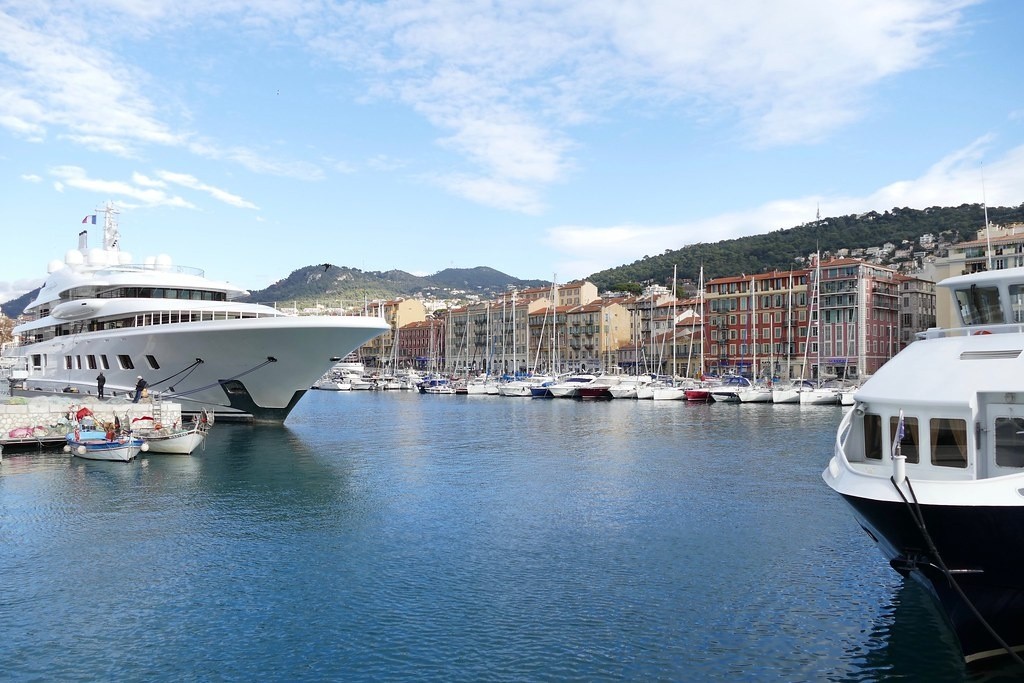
[{"xmin": 137, "ymin": 376, "xmax": 143, "ymax": 379}]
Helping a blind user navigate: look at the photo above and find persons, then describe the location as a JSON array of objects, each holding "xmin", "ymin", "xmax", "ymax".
[
  {"xmin": 96, "ymin": 372, "xmax": 106, "ymax": 398},
  {"xmin": 132, "ymin": 376, "xmax": 147, "ymax": 403}
]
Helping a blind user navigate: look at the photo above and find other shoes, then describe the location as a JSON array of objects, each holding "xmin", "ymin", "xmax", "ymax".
[{"xmin": 132, "ymin": 400, "xmax": 138, "ymax": 403}]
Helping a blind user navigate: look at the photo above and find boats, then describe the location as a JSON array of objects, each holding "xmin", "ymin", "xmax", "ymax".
[
  {"xmin": 64, "ymin": 430, "xmax": 145, "ymax": 462},
  {"xmin": 128, "ymin": 408, "xmax": 215, "ymax": 454},
  {"xmin": 1, "ymin": 198, "xmax": 391, "ymax": 422},
  {"xmin": 821, "ymin": 266, "xmax": 1023, "ymax": 605}
]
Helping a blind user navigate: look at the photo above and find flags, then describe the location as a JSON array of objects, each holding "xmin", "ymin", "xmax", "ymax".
[
  {"xmin": 82, "ymin": 215, "xmax": 96, "ymax": 224},
  {"xmin": 892, "ymin": 408, "xmax": 904, "ymax": 454}
]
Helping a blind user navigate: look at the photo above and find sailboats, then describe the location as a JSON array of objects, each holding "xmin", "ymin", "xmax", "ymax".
[{"xmin": 311, "ymin": 261, "xmax": 865, "ymax": 408}]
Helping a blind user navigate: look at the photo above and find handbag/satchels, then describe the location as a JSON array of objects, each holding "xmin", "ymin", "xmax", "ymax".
[{"xmin": 141, "ymin": 380, "xmax": 148, "ymax": 387}]
